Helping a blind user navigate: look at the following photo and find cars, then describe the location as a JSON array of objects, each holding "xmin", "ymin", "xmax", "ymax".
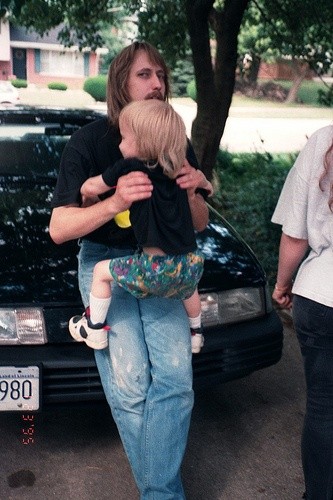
[{"xmin": 0, "ymin": 107, "xmax": 284, "ymax": 414}]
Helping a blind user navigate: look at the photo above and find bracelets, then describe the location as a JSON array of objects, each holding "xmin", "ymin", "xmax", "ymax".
[{"xmin": 275, "ymin": 283, "xmax": 289, "ymax": 291}]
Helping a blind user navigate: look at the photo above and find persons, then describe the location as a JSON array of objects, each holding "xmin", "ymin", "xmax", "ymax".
[
  {"xmin": 270, "ymin": 125, "xmax": 333, "ymax": 500},
  {"xmin": 48, "ymin": 40, "xmax": 222, "ymax": 500},
  {"xmin": 68, "ymin": 99, "xmax": 213, "ymax": 354}
]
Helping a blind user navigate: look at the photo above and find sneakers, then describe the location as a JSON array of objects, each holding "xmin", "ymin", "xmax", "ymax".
[
  {"xmin": 66, "ymin": 309, "xmax": 108, "ymax": 350},
  {"xmin": 190, "ymin": 328, "xmax": 205, "ymax": 355}
]
